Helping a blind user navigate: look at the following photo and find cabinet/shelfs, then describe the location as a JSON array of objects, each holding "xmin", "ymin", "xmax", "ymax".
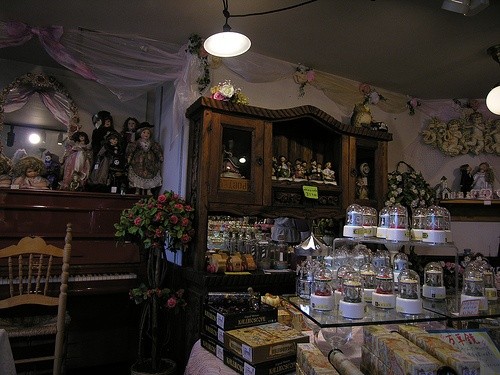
[{"xmin": 183, "ymin": 99, "xmax": 393, "ymax": 365}]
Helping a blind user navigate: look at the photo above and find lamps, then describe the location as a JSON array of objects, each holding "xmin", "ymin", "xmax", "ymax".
[
  {"xmin": 204, "ymin": 0, "xmax": 251, "ymax": 58},
  {"xmin": 56, "ymin": 134, "xmax": 63, "ymax": 145},
  {"xmin": 41, "ymin": 131, "xmax": 47, "ymax": 143},
  {"xmin": 441, "ymin": 0, "xmax": 489, "ymax": 17},
  {"xmin": 6, "ymin": 127, "xmax": 15, "ymax": 146},
  {"xmin": 485, "ymin": 44, "xmax": 500, "ymax": 115}
]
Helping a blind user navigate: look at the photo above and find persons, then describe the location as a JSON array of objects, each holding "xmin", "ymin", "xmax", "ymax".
[
  {"xmin": 63, "ymin": 111, "xmax": 164, "ymax": 195},
  {"xmin": 223, "ymin": 140, "xmax": 240, "ymax": 172},
  {"xmin": 460, "ymin": 253, "xmax": 487, "ymax": 269},
  {"xmin": 0, "ymin": 149, "xmax": 54, "ymax": 188},
  {"xmin": 356, "ymin": 166, "xmax": 368, "ymax": 199},
  {"xmin": 272, "ymin": 156, "xmax": 335, "ymax": 181},
  {"xmin": 460, "ymin": 162, "xmax": 491, "ymax": 198}
]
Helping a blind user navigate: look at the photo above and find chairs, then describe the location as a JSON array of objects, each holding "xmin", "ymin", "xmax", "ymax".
[{"xmin": 0, "ymin": 223, "xmax": 72, "ymax": 375}]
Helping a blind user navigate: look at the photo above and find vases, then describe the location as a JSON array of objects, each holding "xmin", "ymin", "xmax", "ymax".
[{"xmin": 131, "ymin": 358, "xmax": 176, "ymax": 375}]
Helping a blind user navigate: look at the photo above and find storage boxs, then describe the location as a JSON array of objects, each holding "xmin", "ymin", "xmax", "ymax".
[{"xmin": 202, "ymin": 297, "xmax": 460, "ymax": 375}]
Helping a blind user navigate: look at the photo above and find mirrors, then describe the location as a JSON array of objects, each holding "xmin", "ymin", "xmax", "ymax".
[{"xmin": 2, "ymin": 122, "xmax": 68, "ymax": 163}]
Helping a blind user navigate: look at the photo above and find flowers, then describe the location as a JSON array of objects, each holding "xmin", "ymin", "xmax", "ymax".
[
  {"xmin": 387, "ymin": 158, "xmax": 435, "ymax": 209},
  {"xmin": 114, "ymin": 188, "xmax": 194, "ymax": 368}
]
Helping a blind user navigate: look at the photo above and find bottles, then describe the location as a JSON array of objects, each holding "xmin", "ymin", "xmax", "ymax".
[
  {"xmin": 274, "ymin": 234, "xmax": 288, "ymax": 270},
  {"xmin": 207, "ymin": 216, "xmax": 274, "ymax": 271}
]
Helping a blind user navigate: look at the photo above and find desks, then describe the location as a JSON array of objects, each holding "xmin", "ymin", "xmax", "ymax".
[{"xmin": 181, "ymin": 318, "xmax": 456, "ymax": 375}]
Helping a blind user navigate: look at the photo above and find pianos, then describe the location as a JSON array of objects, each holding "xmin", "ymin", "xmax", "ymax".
[{"xmin": 0, "ymin": 186, "xmax": 157, "ymax": 297}]
